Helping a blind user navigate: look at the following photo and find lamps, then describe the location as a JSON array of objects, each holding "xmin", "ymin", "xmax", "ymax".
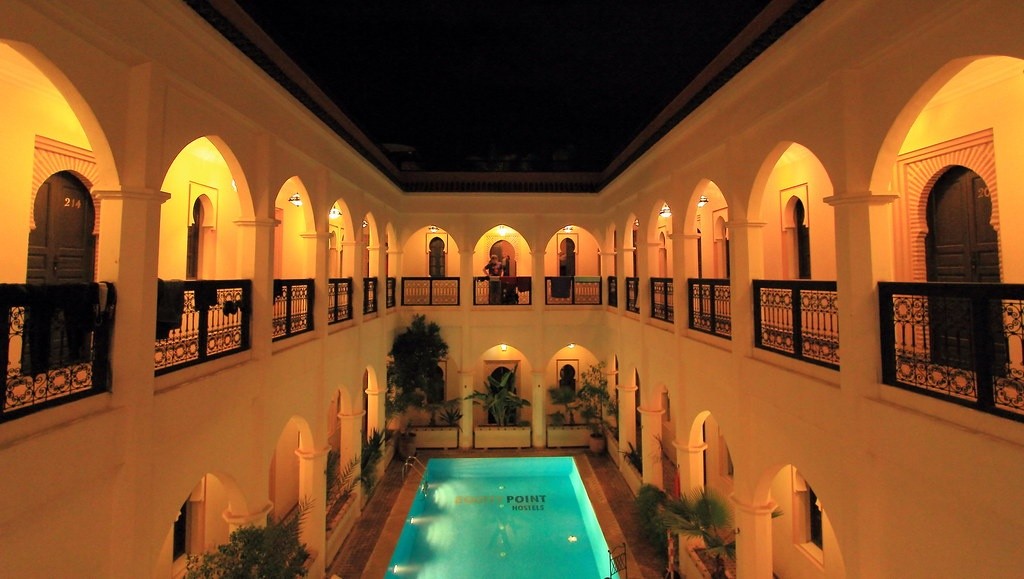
[
  {"xmin": 429, "ymin": 225, "xmax": 439, "ymax": 233},
  {"xmin": 288, "ymin": 192, "xmax": 302, "ymax": 208},
  {"xmin": 329, "ymin": 205, "xmax": 342, "ymax": 218},
  {"xmin": 660, "ymin": 205, "xmax": 671, "ymax": 218},
  {"xmin": 698, "ymin": 195, "xmax": 710, "ymax": 208},
  {"xmin": 563, "ymin": 226, "xmax": 573, "ymax": 233},
  {"xmin": 634, "ymin": 219, "xmax": 639, "ymax": 227},
  {"xmin": 363, "ymin": 220, "xmax": 369, "ymax": 228}
]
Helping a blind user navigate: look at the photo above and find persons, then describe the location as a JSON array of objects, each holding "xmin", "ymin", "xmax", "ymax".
[{"xmin": 483, "ymin": 254, "xmax": 505, "ymax": 305}]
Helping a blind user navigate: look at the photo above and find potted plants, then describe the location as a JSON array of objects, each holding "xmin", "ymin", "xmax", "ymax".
[
  {"xmin": 387, "ymin": 313, "xmax": 449, "ymax": 460},
  {"xmin": 579, "ymin": 360, "xmax": 618, "ymax": 454}
]
